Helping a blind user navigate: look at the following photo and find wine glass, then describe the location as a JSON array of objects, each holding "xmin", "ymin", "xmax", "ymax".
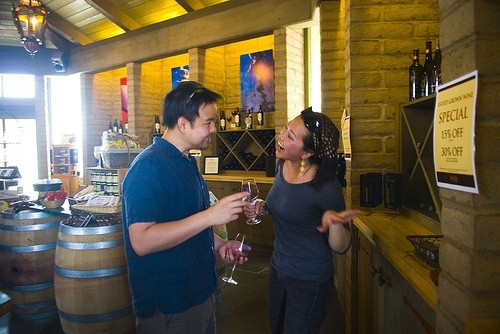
[
  {"xmin": 231, "ymin": 110, "xmax": 235, "ymax": 123},
  {"xmin": 222, "ymin": 233, "xmax": 249, "ymax": 284},
  {"xmin": 241, "ymin": 179, "xmax": 262, "ymax": 224},
  {"xmin": 227, "ymin": 116, "xmax": 231, "ymax": 127}
]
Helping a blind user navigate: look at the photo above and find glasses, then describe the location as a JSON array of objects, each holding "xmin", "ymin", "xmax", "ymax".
[
  {"xmin": 301, "ymin": 106, "xmax": 321, "ymax": 146},
  {"xmin": 185, "ymin": 88, "xmax": 206, "ymax": 106}
]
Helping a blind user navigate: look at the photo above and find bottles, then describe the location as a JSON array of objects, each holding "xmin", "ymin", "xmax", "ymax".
[
  {"xmin": 220, "ymin": 111, "xmax": 226, "ymax": 131},
  {"xmin": 113, "ymin": 119, "xmax": 118, "ymax": 132},
  {"xmin": 108, "ymin": 120, "xmax": 112, "ymax": 132},
  {"xmin": 125, "ymin": 121, "xmax": 128, "ymax": 133},
  {"xmin": 421, "ymin": 41, "xmax": 431, "ymax": 97},
  {"xmin": 155, "ymin": 116, "xmax": 160, "ymax": 133},
  {"xmin": 235, "ymin": 107, "xmax": 240, "ymax": 127},
  {"xmin": 431, "ymin": 38, "xmax": 442, "ymax": 94},
  {"xmin": 409, "ymin": 49, "xmax": 424, "ymax": 101},
  {"xmin": 257, "ymin": 107, "xmax": 263, "ymax": 126},
  {"xmin": 118, "ymin": 121, "xmax": 123, "ymax": 134},
  {"xmin": 246, "ymin": 109, "xmax": 252, "ymax": 129},
  {"xmin": 217, "ymin": 131, "xmax": 274, "ymax": 171}
]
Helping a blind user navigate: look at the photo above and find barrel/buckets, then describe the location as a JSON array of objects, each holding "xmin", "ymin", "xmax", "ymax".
[
  {"xmin": 0, "ymin": 206, "xmax": 66, "ymax": 334},
  {"xmin": 53, "ymin": 215, "xmax": 136, "ymax": 334}
]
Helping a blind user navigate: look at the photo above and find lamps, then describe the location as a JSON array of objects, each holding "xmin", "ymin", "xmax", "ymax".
[{"xmin": 11, "ymin": 0, "xmax": 50, "ymax": 58}]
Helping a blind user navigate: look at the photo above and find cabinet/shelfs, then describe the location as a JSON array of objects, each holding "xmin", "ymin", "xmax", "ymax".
[
  {"xmin": 51, "ymin": 144, "xmax": 79, "ymax": 197},
  {"xmin": 206, "ymin": 180, "xmax": 273, "ymax": 248},
  {"xmin": 215, "ymin": 127, "xmax": 276, "ymax": 173},
  {"xmin": 399, "ymin": 93, "xmax": 442, "ymax": 226},
  {"xmin": 356, "ymin": 229, "xmax": 435, "ymax": 333}
]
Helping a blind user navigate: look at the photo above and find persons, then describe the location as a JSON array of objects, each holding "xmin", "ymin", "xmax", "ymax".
[
  {"xmin": 122, "ymin": 83, "xmax": 252, "ymax": 334},
  {"xmin": 244, "ymin": 106, "xmax": 367, "ymax": 334}
]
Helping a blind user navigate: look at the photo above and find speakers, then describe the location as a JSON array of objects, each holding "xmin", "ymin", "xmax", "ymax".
[{"xmin": 51, "ymin": 51, "xmax": 67, "ymax": 72}]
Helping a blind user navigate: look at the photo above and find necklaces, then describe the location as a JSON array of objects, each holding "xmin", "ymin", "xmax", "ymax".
[{"xmin": 304, "ymin": 164, "xmax": 312, "ymax": 175}]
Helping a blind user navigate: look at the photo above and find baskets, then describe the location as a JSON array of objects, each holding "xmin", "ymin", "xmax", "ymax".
[{"xmin": 97, "ymin": 148, "xmax": 144, "ymax": 169}]
